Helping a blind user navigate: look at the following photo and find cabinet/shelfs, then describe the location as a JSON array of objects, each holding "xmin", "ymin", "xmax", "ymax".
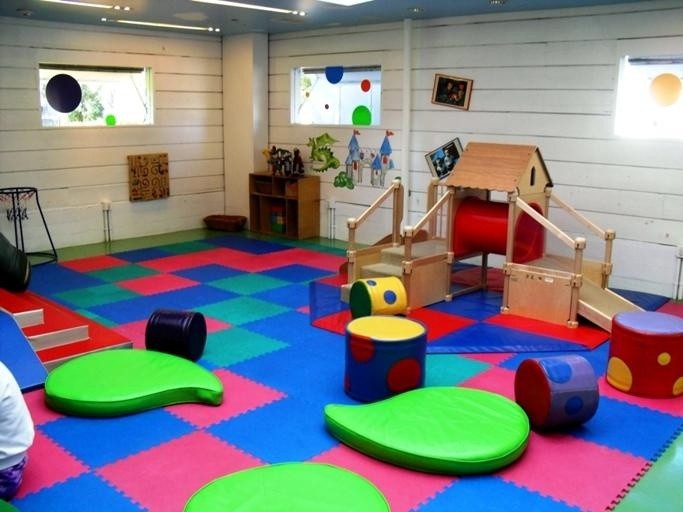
[{"xmin": 247, "ymin": 171, "xmax": 320, "ymax": 241}]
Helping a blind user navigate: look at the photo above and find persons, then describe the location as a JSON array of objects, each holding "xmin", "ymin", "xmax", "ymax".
[
  {"xmin": 444, "ymin": 149, "xmax": 456, "ymax": 171},
  {"xmin": 0, "ymin": 362, "xmax": 35, "ymax": 501},
  {"xmin": 436, "ymin": 158, "xmax": 447, "ymax": 177}
]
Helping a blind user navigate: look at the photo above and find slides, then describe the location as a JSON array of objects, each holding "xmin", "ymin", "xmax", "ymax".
[
  {"xmin": 522, "ymin": 254, "xmax": 645, "ymax": 334},
  {"xmin": 339, "ymin": 230, "xmax": 429, "ymax": 276}
]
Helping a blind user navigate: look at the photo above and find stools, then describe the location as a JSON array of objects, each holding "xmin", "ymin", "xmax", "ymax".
[
  {"xmin": 336, "ymin": 276, "xmax": 428, "ymax": 403},
  {"xmin": 514, "ymin": 311, "xmax": 683, "ymax": 428}
]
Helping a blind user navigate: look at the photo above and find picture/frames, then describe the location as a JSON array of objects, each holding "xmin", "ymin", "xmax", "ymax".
[
  {"xmin": 424, "ymin": 137, "xmax": 463, "ymax": 180},
  {"xmin": 432, "ymin": 73, "xmax": 473, "ymax": 110}
]
